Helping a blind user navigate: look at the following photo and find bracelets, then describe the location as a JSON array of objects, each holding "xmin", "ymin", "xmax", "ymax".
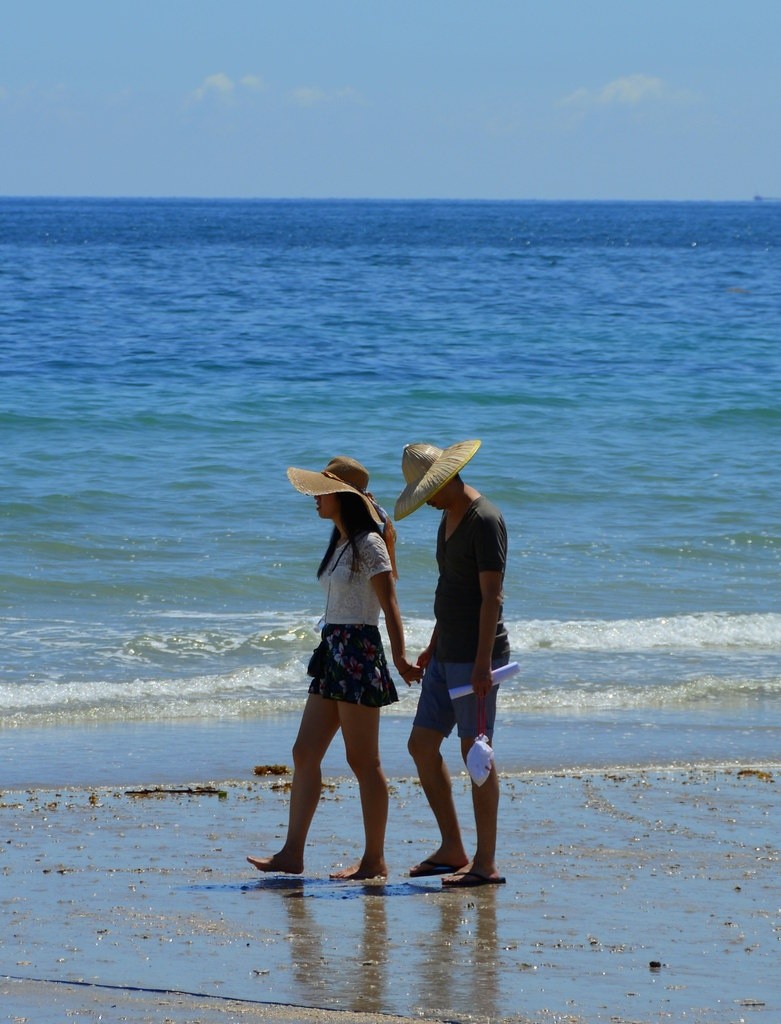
[{"xmin": 398, "ymin": 662, "xmax": 414, "ymax": 675}]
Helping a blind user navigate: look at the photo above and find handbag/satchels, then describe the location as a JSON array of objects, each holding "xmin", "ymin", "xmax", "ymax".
[{"xmin": 468, "ymin": 691, "xmax": 494, "ymax": 787}]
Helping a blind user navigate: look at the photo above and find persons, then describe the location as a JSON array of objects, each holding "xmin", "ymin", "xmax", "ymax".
[
  {"xmin": 244, "ymin": 456, "xmax": 423, "ymax": 880},
  {"xmin": 394, "ymin": 437, "xmax": 512, "ymax": 887}
]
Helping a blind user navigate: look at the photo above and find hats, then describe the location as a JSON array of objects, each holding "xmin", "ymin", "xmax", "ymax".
[
  {"xmin": 287, "ymin": 456, "xmax": 398, "ymax": 581},
  {"xmin": 393, "ymin": 439, "xmax": 483, "ymax": 523}
]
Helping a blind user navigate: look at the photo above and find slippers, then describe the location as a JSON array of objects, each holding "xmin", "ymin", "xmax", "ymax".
[
  {"xmin": 410, "ymin": 854, "xmax": 460, "ymax": 877},
  {"xmin": 442, "ymin": 866, "xmax": 505, "ymax": 887}
]
